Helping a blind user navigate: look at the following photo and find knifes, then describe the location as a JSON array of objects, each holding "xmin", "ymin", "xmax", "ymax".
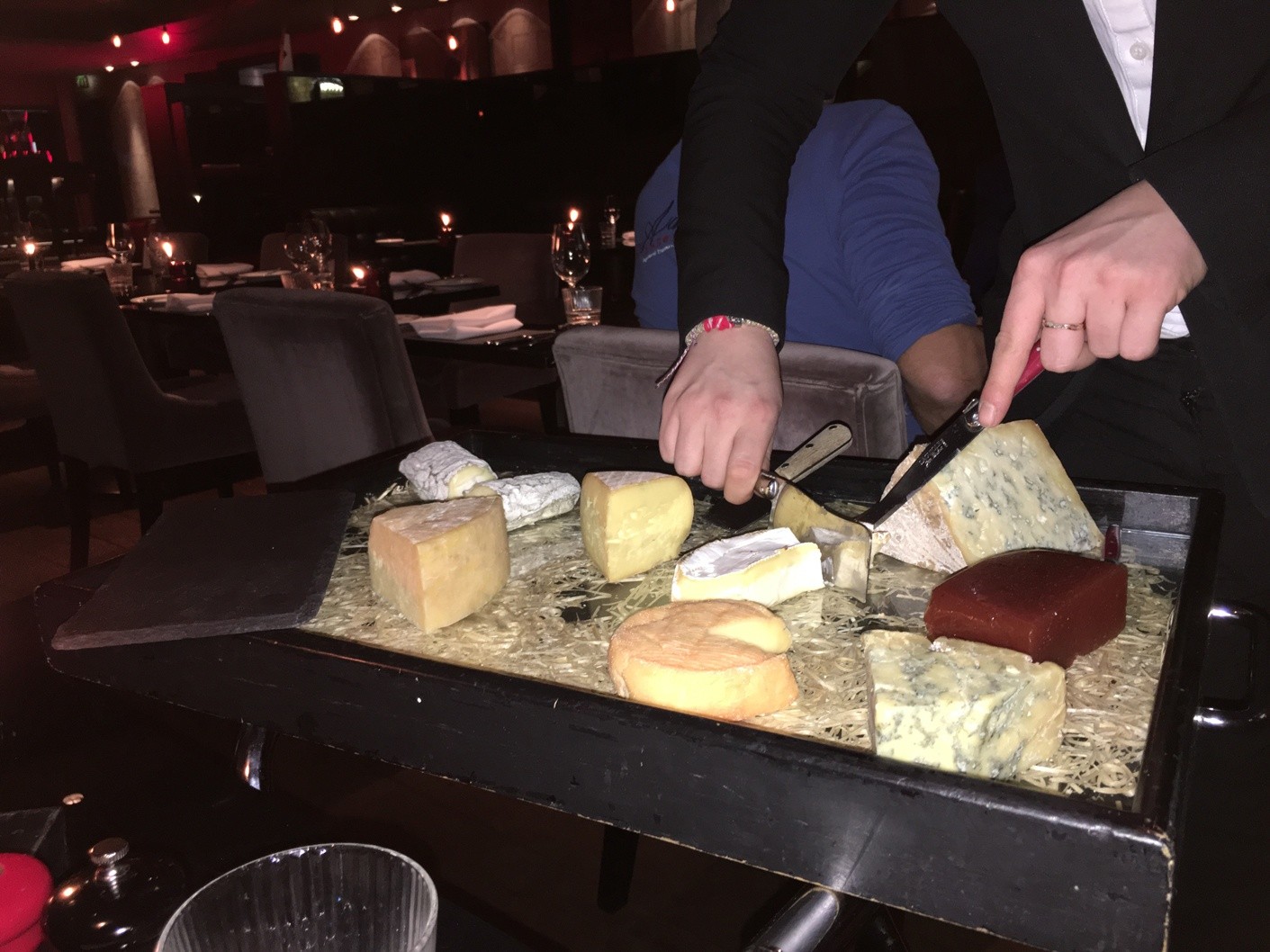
[
  {"xmin": 870, "ymin": 337, "xmax": 1044, "ymax": 528},
  {"xmin": 753, "ymin": 469, "xmax": 872, "ymax": 568}
]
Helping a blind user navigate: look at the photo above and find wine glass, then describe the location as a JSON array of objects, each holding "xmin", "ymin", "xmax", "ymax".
[{"xmin": 551, "ymin": 221, "xmax": 592, "ymax": 290}]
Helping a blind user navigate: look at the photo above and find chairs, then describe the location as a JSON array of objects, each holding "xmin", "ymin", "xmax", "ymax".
[{"xmin": 0, "ymin": 229, "xmax": 906, "ymax": 572}]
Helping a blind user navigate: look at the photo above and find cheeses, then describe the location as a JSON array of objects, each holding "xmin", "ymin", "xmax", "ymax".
[{"xmin": 370, "ymin": 420, "xmax": 1104, "ymax": 779}]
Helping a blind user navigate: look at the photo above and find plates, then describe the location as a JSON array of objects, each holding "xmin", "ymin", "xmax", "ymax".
[
  {"xmin": 240, "ymin": 269, "xmax": 293, "ymax": 283},
  {"xmin": 427, "ymin": 276, "xmax": 484, "ymax": 293},
  {"xmin": 129, "ymin": 292, "xmax": 200, "ymax": 305}
]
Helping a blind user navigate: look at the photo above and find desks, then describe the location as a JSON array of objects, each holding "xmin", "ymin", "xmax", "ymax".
[
  {"xmin": 368, "ymin": 234, "xmax": 458, "ymax": 296},
  {"xmin": 584, "ymin": 237, "xmax": 636, "ymax": 254},
  {"xmin": 117, "ymin": 294, "xmax": 567, "ymax": 435},
  {"xmin": 1, "ymin": 255, "xmax": 501, "ymax": 315}
]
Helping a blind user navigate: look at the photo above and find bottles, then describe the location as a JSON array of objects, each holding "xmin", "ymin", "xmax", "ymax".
[{"xmin": 0, "ymin": 837, "xmax": 158, "ymax": 952}]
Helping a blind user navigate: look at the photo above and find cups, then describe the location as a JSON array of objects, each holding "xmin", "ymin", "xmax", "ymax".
[
  {"xmin": 153, "ymin": 842, "xmax": 441, "ymax": 952},
  {"xmin": 598, "ymin": 194, "xmax": 622, "ymax": 249},
  {"xmin": 105, "ymin": 263, "xmax": 133, "ymax": 294},
  {"xmin": 105, "ymin": 222, "xmax": 134, "ymax": 262},
  {"xmin": 561, "ymin": 285, "xmax": 603, "ymax": 327},
  {"xmin": 285, "ymin": 230, "xmax": 335, "ymax": 291}
]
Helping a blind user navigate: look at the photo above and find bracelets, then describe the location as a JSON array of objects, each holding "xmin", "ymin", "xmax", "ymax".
[{"xmin": 654, "ymin": 313, "xmax": 781, "ymax": 389}]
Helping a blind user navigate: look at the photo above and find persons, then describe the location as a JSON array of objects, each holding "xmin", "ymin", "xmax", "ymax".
[
  {"xmin": 658, "ymin": 2, "xmax": 1270, "ymax": 952},
  {"xmin": 625, "ymin": 95, "xmax": 984, "ymax": 447}
]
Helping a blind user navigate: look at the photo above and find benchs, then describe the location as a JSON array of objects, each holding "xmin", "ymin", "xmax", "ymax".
[{"xmin": 305, "ymin": 202, "xmax": 448, "ymax": 298}]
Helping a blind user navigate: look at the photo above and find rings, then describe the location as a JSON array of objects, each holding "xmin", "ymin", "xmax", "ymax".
[{"xmin": 1042, "ymin": 318, "xmax": 1084, "ymax": 331}]
